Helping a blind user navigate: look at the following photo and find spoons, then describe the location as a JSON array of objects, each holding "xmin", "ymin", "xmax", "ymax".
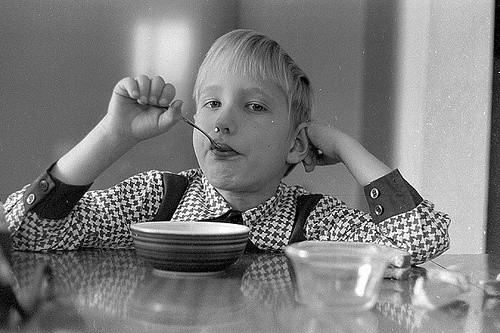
[{"xmin": 133, "ymin": 100, "xmax": 230, "ymax": 152}]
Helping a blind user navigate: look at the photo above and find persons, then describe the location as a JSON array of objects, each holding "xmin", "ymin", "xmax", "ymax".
[{"xmin": 3, "ymin": 29, "xmax": 451, "ymax": 265}]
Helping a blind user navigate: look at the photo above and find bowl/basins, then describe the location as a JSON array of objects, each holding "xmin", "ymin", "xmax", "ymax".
[
  {"xmin": 284, "ymin": 241, "xmax": 393, "ymax": 312},
  {"xmin": 129, "ymin": 221, "xmax": 250, "ymax": 275}
]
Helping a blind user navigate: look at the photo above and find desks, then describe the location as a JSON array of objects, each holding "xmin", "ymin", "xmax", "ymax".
[{"xmin": 0, "ymin": 249, "xmax": 500, "ymax": 333}]
